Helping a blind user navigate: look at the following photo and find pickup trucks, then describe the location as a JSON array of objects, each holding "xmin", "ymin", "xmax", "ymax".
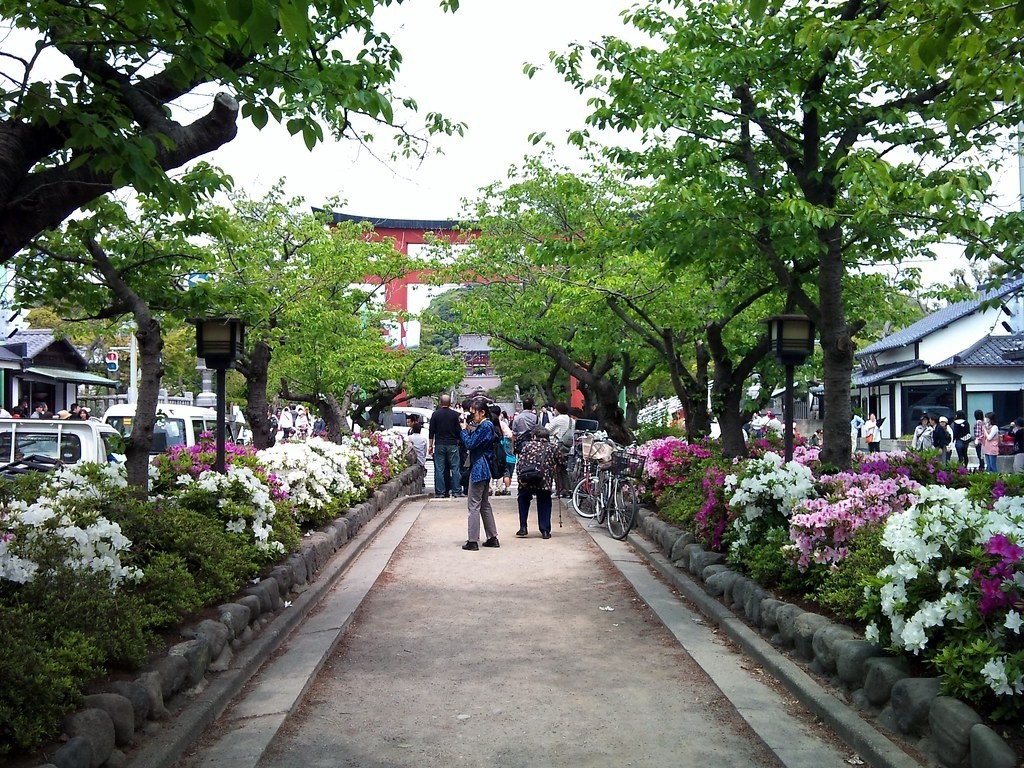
[{"xmin": 0, "ymin": 417, "xmax": 124, "ymax": 495}]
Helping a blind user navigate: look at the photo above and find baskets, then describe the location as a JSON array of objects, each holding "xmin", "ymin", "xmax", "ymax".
[{"xmin": 610, "ymin": 451, "xmax": 647, "ymax": 478}]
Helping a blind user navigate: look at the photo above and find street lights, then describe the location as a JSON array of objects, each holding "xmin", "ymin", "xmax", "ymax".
[
  {"xmin": 758, "ymin": 311, "xmax": 817, "ymax": 468},
  {"xmin": 184, "ymin": 314, "xmax": 247, "ymax": 474}
]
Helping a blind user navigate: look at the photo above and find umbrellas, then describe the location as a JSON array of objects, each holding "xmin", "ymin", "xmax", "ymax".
[{"xmin": 876, "ymin": 417, "xmax": 886, "ymax": 428}]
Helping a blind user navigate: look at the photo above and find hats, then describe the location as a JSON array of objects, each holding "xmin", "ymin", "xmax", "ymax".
[
  {"xmin": 921, "ymin": 416, "xmax": 928, "ymax": 421},
  {"xmin": 939, "ymin": 416, "xmax": 949, "ymax": 423},
  {"xmin": 554, "ymin": 401, "xmax": 567, "ymax": 414},
  {"xmin": 1014, "ymin": 417, "xmax": 1024, "ymax": 426}
]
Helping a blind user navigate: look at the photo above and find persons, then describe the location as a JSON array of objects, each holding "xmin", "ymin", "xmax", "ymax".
[
  {"xmin": 809, "ymin": 429, "xmax": 823, "ymax": 446},
  {"xmin": 973, "ymin": 410, "xmax": 998, "ymax": 473},
  {"xmin": 911, "ymin": 414, "xmax": 954, "ymax": 465},
  {"xmin": 0, "ymin": 398, "xmax": 93, "ymax": 421},
  {"xmin": 1007, "ymin": 417, "xmax": 1024, "ymax": 473},
  {"xmin": 864, "ymin": 414, "xmax": 883, "ymax": 453},
  {"xmin": 270, "ymin": 404, "xmax": 325, "ymax": 439},
  {"xmin": 850, "ymin": 409, "xmax": 865, "ymax": 454},
  {"xmin": 710, "ymin": 411, "xmax": 799, "ymax": 442},
  {"xmin": 513, "ymin": 398, "xmax": 576, "ymax": 539},
  {"xmin": 428, "ymin": 395, "xmax": 516, "ymax": 551},
  {"xmin": 408, "ymin": 414, "xmax": 427, "ymax": 489},
  {"xmin": 950, "ymin": 410, "xmax": 970, "ymax": 469}
]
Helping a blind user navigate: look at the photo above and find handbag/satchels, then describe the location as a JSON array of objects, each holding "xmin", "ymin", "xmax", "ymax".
[
  {"xmin": 513, "ymin": 430, "xmax": 534, "ymax": 454},
  {"xmin": 864, "ymin": 434, "xmax": 873, "ymax": 442},
  {"xmin": 558, "ymin": 429, "xmax": 574, "ymax": 453},
  {"xmin": 520, "ymin": 465, "xmax": 544, "ymax": 482},
  {"xmin": 959, "ymin": 434, "xmax": 972, "ymax": 443}
]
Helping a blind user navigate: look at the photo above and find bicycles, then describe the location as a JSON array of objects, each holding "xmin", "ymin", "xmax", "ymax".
[{"xmin": 572, "ymin": 436, "xmax": 647, "ymax": 541}]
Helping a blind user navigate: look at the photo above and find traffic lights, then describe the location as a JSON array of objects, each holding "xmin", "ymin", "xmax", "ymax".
[{"xmin": 105, "ymin": 351, "xmax": 118, "ymax": 371}]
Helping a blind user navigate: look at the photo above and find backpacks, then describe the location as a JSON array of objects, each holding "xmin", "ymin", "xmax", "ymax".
[{"xmin": 477, "ymin": 424, "xmax": 507, "ymax": 479}]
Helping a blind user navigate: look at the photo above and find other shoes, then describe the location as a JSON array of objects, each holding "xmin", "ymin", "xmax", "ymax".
[
  {"xmin": 434, "ymin": 489, "xmax": 511, "ymax": 498},
  {"xmin": 516, "ymin": 527, "xmax": 529, "ymax": 536},
  {"xmin": 541, "ymin": 530, "xmax": 552, "ymax": 539}
]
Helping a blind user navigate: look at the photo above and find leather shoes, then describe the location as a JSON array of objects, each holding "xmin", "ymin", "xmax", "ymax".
[
  {"xmin": 482, "ymin": 538, "xmax": 500, "ymax": 548},
  {"xmin": 462, "ymin": 540, "xmax": 478, "ymax": 551}
]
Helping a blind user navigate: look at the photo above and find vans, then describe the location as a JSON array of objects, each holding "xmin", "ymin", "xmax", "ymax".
[
  {"xmin": 349, "ymin": 407, "xmax": 435, "ymax": 458},
  {"xmin": 101, "ymin": 403, "xmax": 244, "ymax": 475}
]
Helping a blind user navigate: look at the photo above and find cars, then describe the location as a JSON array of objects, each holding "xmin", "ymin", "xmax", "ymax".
[{"xmin": 996, "ymin": 423, "xmax": 1024, "ymax": 454}]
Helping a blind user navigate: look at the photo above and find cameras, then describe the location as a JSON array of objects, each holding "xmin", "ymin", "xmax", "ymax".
[{"xmin": 458, "ymin": 413, "xmax": 474, "ymax": 424}]
